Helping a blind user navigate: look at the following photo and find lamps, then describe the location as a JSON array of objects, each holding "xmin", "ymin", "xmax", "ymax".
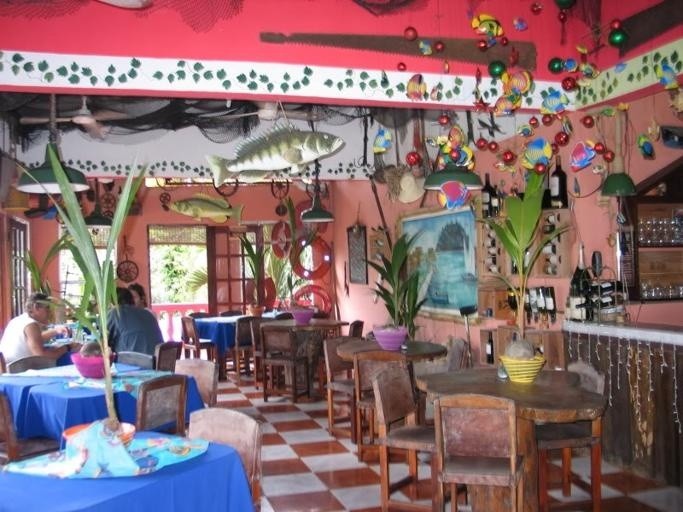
[
  {"xmin": 602, "ymin": 109, "xmax": 634, "ymax": 199},
  {"xmin": 83, "ymin": 181, "xmax": 113, "ymax": 231},
  {"xmin": 297, "ymin": 117, "xmax": 332, "ymax": 223},
  {"xmin": 22, "ymin": 92, "xmax": 87, "ymax": 194},
  {"xmin": 423, "ymin": 167, "xmax": 482, "ymax": 190}
]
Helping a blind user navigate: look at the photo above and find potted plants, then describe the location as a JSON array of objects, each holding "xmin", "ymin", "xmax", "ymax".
[
  {"xmin": 235, "ymin": 233, "xmax": 275, "ymax": 316},
  {"xmin": 10, "ymin": 138, "xmax": 147, "ymax": 383},
  {"xmin": 14, "ymin": 147, "xmax": 135, "ymax": 446},
  {"xmin": 284, "ymin": 195, "xmax": 314, "ymax": 324},
  {"xmin": 471, "ymin": 161, "xmax": 572, "ymax": 383},
  {"xmin": 361, "ymin": 228, "xmax": 423, "ymax": 352}
]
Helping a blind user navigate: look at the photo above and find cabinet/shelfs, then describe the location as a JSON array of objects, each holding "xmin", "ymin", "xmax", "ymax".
[
  {"xmin": 612, "ymin": 156, "xmax": 683, "ymax": 302},
  {"xmin": 475, "ymin": 204, "xmax": 572, "ymax": 373}
]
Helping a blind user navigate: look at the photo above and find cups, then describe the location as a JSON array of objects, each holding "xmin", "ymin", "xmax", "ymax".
[{"xmin": 635, "ymin": 215, "xmax": 683, "ymax": 300}]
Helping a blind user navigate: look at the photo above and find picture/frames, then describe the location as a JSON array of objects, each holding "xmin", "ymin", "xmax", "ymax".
[
  {"xmin": 347, "ymin": 224, "xmax": 368, "ymax": 284},
  {"xmin": 393, "ymin": 201, "xmax": 480, "ymax": 325}
]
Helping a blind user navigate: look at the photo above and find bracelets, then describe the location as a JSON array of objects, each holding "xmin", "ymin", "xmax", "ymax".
[{"xmin": 66, "ymin": 344, "xmax": 70, "ymax": 351}]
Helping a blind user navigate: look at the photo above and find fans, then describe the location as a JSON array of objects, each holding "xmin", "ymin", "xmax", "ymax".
[
  {"xmin": 199, "ymin": 100, "xmax": 322, "ymax": 126},
  {"xmin": 17, "ymin": 93, "xmax": 124, "ymax": 142}
]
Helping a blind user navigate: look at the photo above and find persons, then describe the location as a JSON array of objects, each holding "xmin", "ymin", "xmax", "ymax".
[
  {"xmin": 0, "ymin": 292, "xmax": 79, "ymax": 372},
  {"xmin": 102, "ymin": 287, "xmax": 164, "ymax": 355},
  {"xmin": 128, "ymin": 284, "xmax": 156, "ymax": 318}
]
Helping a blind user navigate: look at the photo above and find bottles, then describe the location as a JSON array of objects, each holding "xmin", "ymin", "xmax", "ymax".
[
  {"xmin": 549, "ymin": 154, "xmax": 568, "ymax": 209},
  {"xmin": 485, "ymin": 332, "xmax": 494, "ymax": 365},
  {"xmin": 490, "ymin": 184, "xmax": 499, "ymax": 216},
  {"xmin": 507, "ymin": 286, "xmax": 555, "ymax": 324},
  {"xmin": 575, "ymin": 300, "xmax": 593, "ymax": 310},
  {"xmin": 480, "ymin": 172, "xmax": 493, "ymax": 218},
  {"xmin": 569, "ymin": 242, "xmax": 623, "ymax": 321},
  {"xmin": 481, "ymin": 212, "xmax": 562, "ymax": 275}
]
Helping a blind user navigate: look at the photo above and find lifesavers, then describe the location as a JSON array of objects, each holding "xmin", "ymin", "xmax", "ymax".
[
  {"xmin": 272, "ymin": 222, "xmax": 291, "ymax": 257},
  {"xmin": 294, "ymin": 200, "xmax": 327, "ymax": 236},
  {"xmin": 295, "ymin": 285, "xmax": 332, "ymax": 318},
  {"xmin": 289, "ymin": 233, "xmax": 332, "ymax": 280},
  {"xmin": 245, "ymin": 272, "xmax": 276, "ymax": 313}
]
